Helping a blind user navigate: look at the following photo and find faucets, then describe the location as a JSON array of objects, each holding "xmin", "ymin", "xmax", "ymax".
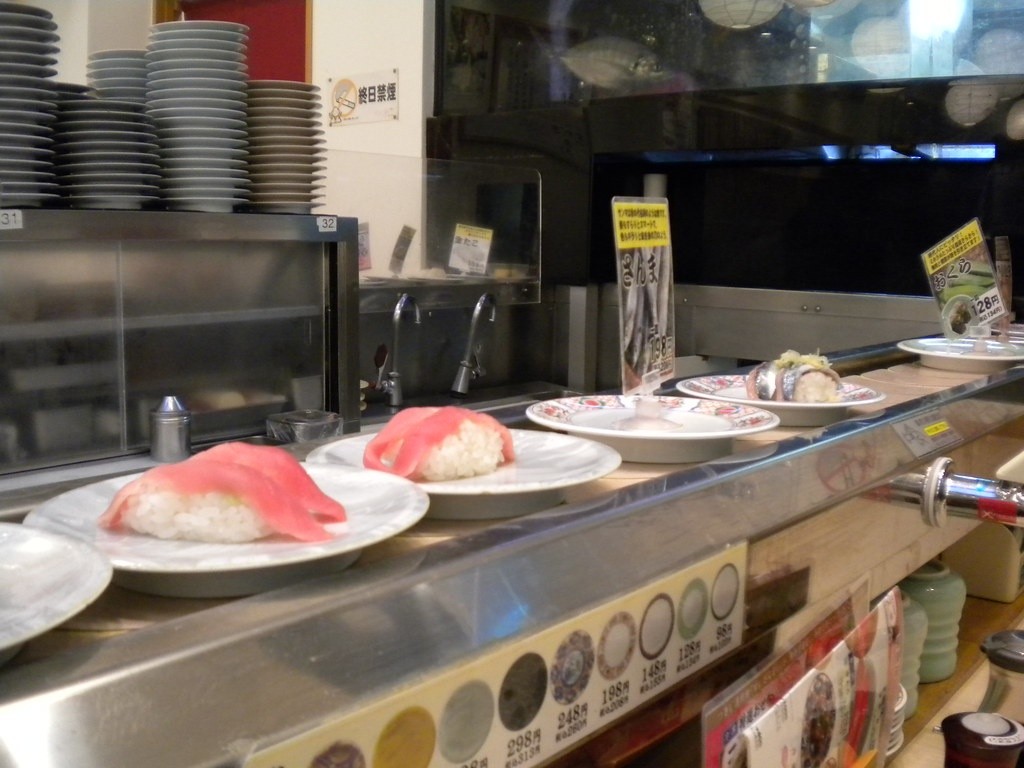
[
  {"xmin": 451, "ymin": 292, "xmax": 497, "ymax": 393},
  {"xmin": 371, "ymin": 295, "xmax": 421, "ymax": 405}
]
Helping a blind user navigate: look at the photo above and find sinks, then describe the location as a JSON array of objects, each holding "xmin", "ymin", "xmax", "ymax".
[{"xmin": 361, "ymin": 389, "xmax": 584, "ymax": 426}]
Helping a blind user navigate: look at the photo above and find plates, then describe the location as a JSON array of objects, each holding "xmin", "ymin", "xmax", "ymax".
[
  {"xmin": 677, "ymin": 578, "xmax": 708, "ymax": 639},
  {"xmin": 711, "ymin": 563, "xmax": 740, "ymax": 619},
  {"xmin": 21, "ymin": 462, "xmax": 430, "ymax": 597},
  {"xmin": 359, "ymin": 273, "xmax": 536, "ymax": 287},
  {"xmin": 596, "ymin": 612, "xmax": 636, "ymax": 679},
  {"xmin": 372, "ymin": 707, "xmax": 436, "ymax": 768},
  {"xmin": 525, "ymin": 394, "xmax": 780, "ymax": 462},
  {"xmin": 498, "ymin": 653, "xmax": 547, "ymax": 731},
  {"xmin": 0, "ymin": 2, "xmax": 327, "ymax": 215},
  {"xmin": 305, "ymin": 428, "xmax": 622, "ymax": 519},
  {"xmin": 886, "ymin": 683, "xmax": 907, "ymax": 758},
  {"xmin": 549, "ymin": 629, "xmax": 594, "ymax": 704},
  {"xmin": 639, "ymin": 594, "xmax": 675, "ymax": 660},
  {"xmin": 941, "ymin": 295, "xmax": 979, "ymax": 342},
  {"xmin": 310, "ymin": 741, "xmax": 366, "ymax": 768},
  {"xmin": 438, "ymin": 681, "xmax": 494, "ymax": 763},
  {"xmin": 991, "ymin": 324, "xmax": 1024, "ymax": 337},
  {"xmin": 896, "ymin": 338, "xmax": 1024, "ymax": 374},
  {"xmin": 0, "ymin": 522, "xmax": 113, "ymax": 665},
  {"xmin": 675, "ymin": 374, "xmax": 886, "ymax": 426}
]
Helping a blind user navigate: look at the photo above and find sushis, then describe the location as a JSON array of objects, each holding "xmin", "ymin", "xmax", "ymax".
[
  {"xmin": 362, "ymin": 405, "xmax": 517, "ymax": 482},
  {"xmin": 98, "ymin": 442, "xmax": 347, "ymax": 544},
  {"xmin": 746, "ymin": 358, "xmax": 844, "ymax": 404}
]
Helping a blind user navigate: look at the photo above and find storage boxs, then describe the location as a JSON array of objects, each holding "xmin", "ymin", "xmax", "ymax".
[{"xmin": 940, "ymin": 521, "xmax": 1024, "ymax": 603}]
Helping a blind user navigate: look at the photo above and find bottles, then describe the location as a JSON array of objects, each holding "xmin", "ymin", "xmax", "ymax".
[
  {"xmin": 895, "ymin": 559, "xmax": 967, "ymax": 683},
  {"xmin": 869, "ymin": 588, "xmax": 927, "ymax": 720},
  {"xmin": 977, "ymin": 630, "xmax": 1024, "ymax": 726},
  {"xmin": 941, "ymin": 712, "xmax": 1024, "ymax": 768}
]
